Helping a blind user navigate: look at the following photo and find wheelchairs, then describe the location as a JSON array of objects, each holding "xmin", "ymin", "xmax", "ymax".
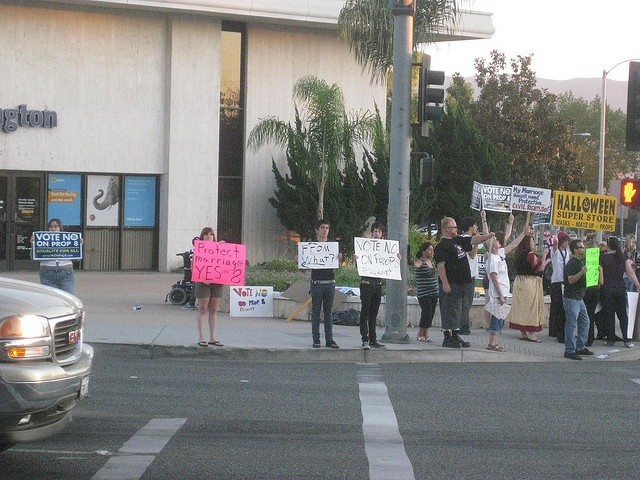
[{"xmin": 165, "ymin": 250, "xmax": 198, "ymax": 306}]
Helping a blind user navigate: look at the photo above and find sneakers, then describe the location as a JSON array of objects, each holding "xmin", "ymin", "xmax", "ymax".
[
  {"xmin": 313, "ymin": 340, "xmax": 321, "ymax": 347},
  {"xmin": 441, "ymin": 337, "xmax": 462, "ymax": 349},
  {"xmin": 626, "ymin": 343, "xmax": 635, "ymax": 348},
  {"xmin": 362, "ymin": 340, "xmax": 371, "ymax": 351},
  {"xmin": 369, "ymin": 341, "xmax": 386, "ymax": 349},
  {"xmin": 326, "ymin": 340, "xmax": 340, "ymax": 349},
  {"xmin": 604, "ymin": 342, "xmax": 615, "ymax": 347},
  {"xmin": 453, "ymin": 336, "xmax": 471, "ymax": 348}
]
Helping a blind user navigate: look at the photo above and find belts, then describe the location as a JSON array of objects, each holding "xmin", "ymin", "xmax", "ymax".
[
  {"xmin": 311, "ymin": 280, "xmax": 335, "ymax": 285},
  {"xmin": 361, "ymin": 280, "xmax": 382, "ymax": 286}
]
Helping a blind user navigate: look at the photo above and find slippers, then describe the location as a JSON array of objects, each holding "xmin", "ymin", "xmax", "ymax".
[
  {"xmin": 417, "ymin": 336, "xmax": 427, "ymax": 342},
  {"xmin": 208, "ymin": 341, "xmax": 225, "ymax": 346},
  {"xmin": 426, "ymin": 337, "xmax": 434, "ymax": 343},
  {"xmin": 519, "ymin": 334, "xmax": 528, "ymax": 340},
  {"xmin": 527, "ymin": 337, "xmax": 542, "ymax": 343},
  {"xmin": 198, "ymin": 341, "xmax": 208, "ymax": 347}
]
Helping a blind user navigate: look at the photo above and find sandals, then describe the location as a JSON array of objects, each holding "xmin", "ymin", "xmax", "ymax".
[{"xmin": 487, "ymin": 344, "xmax": 504, "ymax": 352}]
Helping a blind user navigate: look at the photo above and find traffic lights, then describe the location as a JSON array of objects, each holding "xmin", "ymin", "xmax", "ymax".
[
  {"xmin": 621, "ymin": 177, "xmax": 638, "ymax": 209},
  {"xmin": 422, "ymin": 67, "xmax": 446, "ymax": 124}
]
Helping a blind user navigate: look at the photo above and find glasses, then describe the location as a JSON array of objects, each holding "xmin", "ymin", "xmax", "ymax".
[
  {"xmin": 576, "ymin": 246, "xmax": 585, "ymax": 249},
  {"xmin": 607, "ymin": 236, "xmax": 617, "ymax": 242},
  {"xmin": 449, "ymin": 225, "xmax": 457, "ymax": 229},
  {"xmin": 48, "ymin": 225, "xmax": 59, "ymax": 228},
  {"xmin": 319, "ymin": 226, "xmax": 329, "ymax": 230}
]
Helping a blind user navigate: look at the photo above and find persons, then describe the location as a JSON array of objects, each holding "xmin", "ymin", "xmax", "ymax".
[
  {"xmin": 595, "ymin": 241, "xmax": 609, "ymax": 339},
  {"xmin": 598, "ymin": 237, "xmax": 640, "ymax": 348},
  {"xmin": 494, "ymin": 214, "xmax": 515, "ymax": 292},
  {"xmin": 301, "ymin": 219, "xmax": 344, "ymax": 349},
  {"xmin": 180, "ymin": 283, "xmax": 196, "ymax": 310},
  {"xmin": 563, "ymin": 239, "xmax": 594, "ymax": 360},
  {"xmin": 622, "ymin": 235, "xmax": 640, "ymax": 293},
  {"xmin": 514, "ymin": 236, "xmax": 549, "ymax": 343},
  {"xmin": 408, "ymin": 242, "xmax": 440, "ymax": 343},
  {"xmin": 582, "ymin": 240, "xmax": 596, "ymax": 347},
  {"xmin": 30, "ymin": 218, "xmax": 84, "ymax": 296},
  {"xmin": 434, "ymin": 217, "xmax": 496, "ymax": 349},
  {"xmin": 550, "ymin": 232, "xmax": 569, "ymax": 343},
  {"xmin": 353, "ymin": 222, "xmax": 402, "ymax": 351},
  {"xmin": 541, "ymin": 238, "xmax": 554, "ymax": 296},
  {"xmin": 190, "ymin": 227, "xmax": 226, "ymax": 347},
  {"xmin": 457, "ymin": 211, "xmax": 488, "ymax": 335},
  {"xmin": 189, "ymin": 236, "xmax": 200, "ymax": 261},
  {"xmin": 484, "ymin": 225, "xmax": 530, "ymax": 351}
]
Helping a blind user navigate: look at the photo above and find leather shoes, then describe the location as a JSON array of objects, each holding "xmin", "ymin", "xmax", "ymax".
[
  {"xmin": 564, "ymin": 352, "xmax": 583, "ymax": 361},
  {"xmin": 576, "ymin": 348, "xmax": 594, "ymax": 356}
]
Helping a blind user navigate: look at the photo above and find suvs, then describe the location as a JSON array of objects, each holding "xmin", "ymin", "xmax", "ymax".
[{"xmin": 0, "ymin": 275, "xmax": 93, "ymax": 456}]
